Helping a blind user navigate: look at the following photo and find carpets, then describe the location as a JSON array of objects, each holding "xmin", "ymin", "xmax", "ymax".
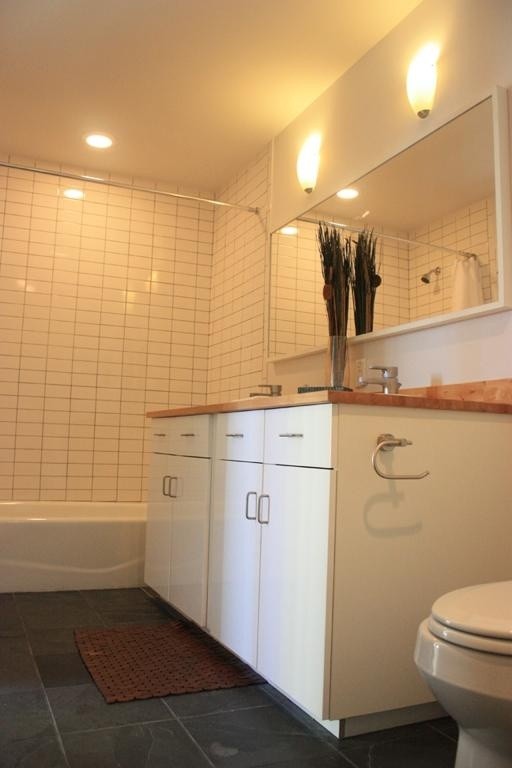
[{"xmin": 70, "ymin": 622, "xmax": 268, "ymax": 706}]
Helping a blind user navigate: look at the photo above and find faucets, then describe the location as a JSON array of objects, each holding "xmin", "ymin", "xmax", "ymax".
[
  {"xmin": 354, "ymin": 364, "xmax": 402, "ymax": 395},
  {"xmin": 248, "ymin": 384, "xmax": 282, "ymax": 397}
]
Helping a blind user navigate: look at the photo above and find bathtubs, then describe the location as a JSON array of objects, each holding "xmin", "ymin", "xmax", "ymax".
[{"xmin": 1, "ymin": 500, "xmax": 148, "ymax": 594}]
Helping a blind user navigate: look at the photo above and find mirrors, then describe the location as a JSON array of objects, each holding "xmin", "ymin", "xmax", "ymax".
[{"xmin": 262, "ymin": 85, "xmax": 510, "ymax": 365}]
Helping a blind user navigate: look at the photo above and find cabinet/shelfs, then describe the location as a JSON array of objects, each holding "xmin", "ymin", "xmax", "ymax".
[
  {"xmin": 212, "ymin": 401, "xmax": 512, "ymax": 743},
  {"xmin": 142, "ymin": 405, "xmax": 209, "ymax": 647}
]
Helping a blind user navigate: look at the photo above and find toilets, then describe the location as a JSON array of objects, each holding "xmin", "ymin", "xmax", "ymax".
[{"xmin": 414, "ymin": 580, "xmax": 511, "ymax": 767}]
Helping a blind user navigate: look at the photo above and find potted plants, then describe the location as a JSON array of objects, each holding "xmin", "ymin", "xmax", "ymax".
[
  {"xmin": 351, "ymin": 227, "xmax": 379, "ymax": 336},
  {"xmin": 314, "ymin": 221, "xmax": 356, "ymax": 387}
]
